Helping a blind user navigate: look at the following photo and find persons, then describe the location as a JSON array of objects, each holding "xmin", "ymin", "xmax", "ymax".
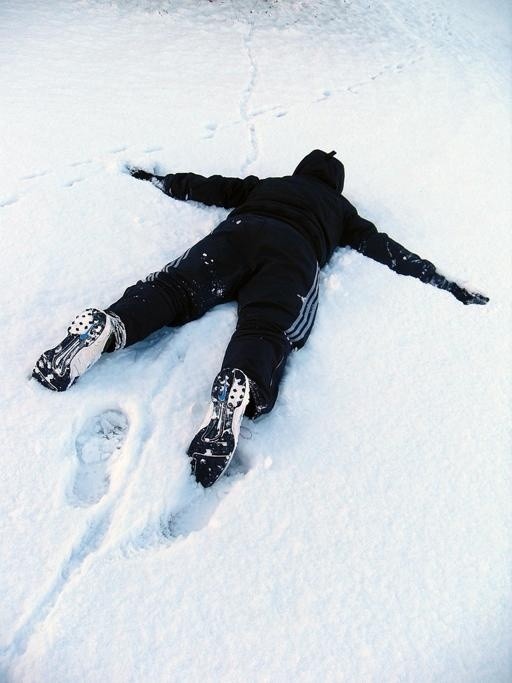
[{"xmin": 33, "ymin": 149, "xmax": 490, "ymax": 491}]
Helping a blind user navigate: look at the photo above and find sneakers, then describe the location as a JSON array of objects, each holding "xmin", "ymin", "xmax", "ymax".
[
  {"xmin": 32, "ymin": 307, "xmax": 115, "ymax": 392},
  {"xmin": 186, "ymin": 366, "xmax": 253, "ymax": 488}
]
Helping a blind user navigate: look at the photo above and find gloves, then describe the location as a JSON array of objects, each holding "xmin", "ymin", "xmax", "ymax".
[
  {"xmin": 128, "ymin": 166, "xmax": 161, "ymax": 182},
  {"xmin": 445, "ymin": 280, "xmax": 489, "ymax": 305}
]
